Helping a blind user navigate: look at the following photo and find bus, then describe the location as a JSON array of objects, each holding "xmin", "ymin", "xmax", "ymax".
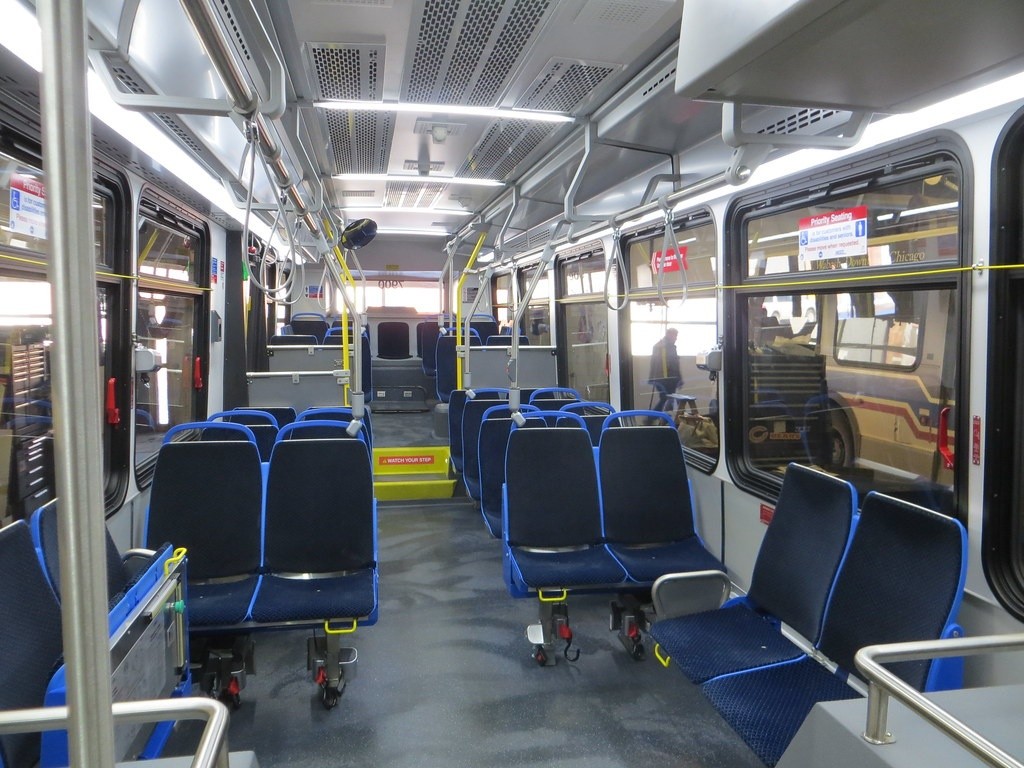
[{"xmin": 746, "ymin": 204, "xmax": 956, "ymax": 490}]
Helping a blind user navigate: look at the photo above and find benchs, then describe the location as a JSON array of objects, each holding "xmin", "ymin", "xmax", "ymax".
[{"xmin": 0, "ymin": 312, "xmax": 969, "ymax": 768}]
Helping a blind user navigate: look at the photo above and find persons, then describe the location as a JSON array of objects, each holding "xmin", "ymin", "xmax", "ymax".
[
  {"xmin": 641, "ymin": 328, "xmax": 684, "ymax": 426},
  {"xmin": 762, "ymin": 306, "xmax": 816, "ymax": 325}
]
